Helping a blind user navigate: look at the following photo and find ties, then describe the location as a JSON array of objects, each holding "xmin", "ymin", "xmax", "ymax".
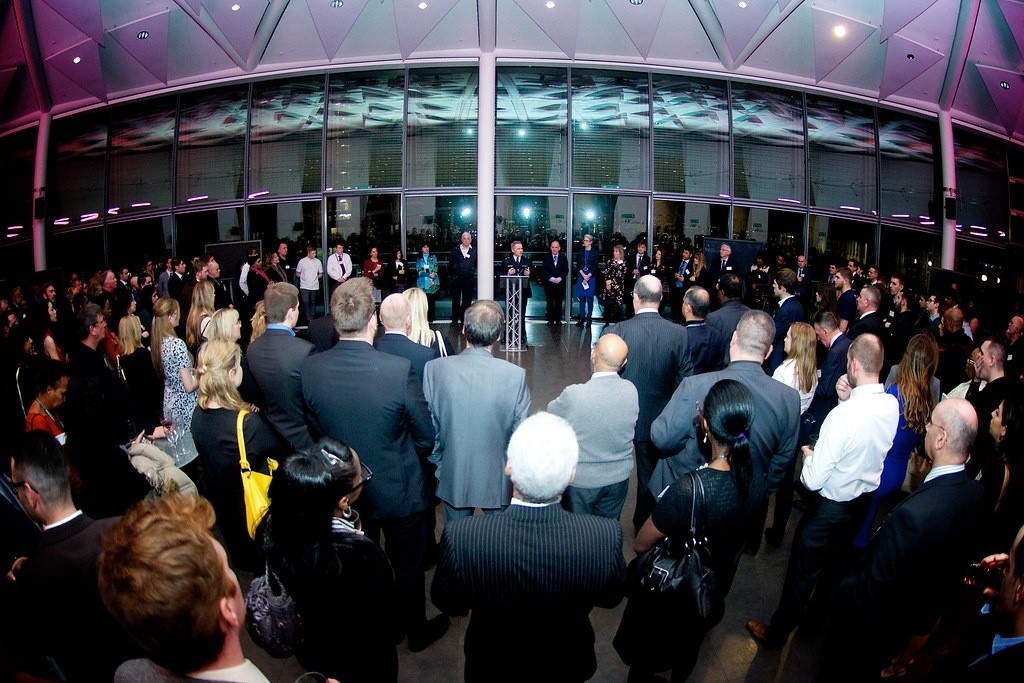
[
  {"xmin": 339, "ymin": 257, "xmax": 346, "ymax": 277},
  {"xmin": 680, "ymin": 261, "xmax": 685, "ymax": 274},
  {"xmin": 722, "ymin": 260, "xmax": 725, "ymax": 268},
  {"xmin": 799, "ymin": 269, "xmax": 802, "ymax": 277}
]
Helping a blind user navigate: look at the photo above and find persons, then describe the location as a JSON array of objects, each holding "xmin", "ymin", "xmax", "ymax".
[{"xmin": 0, "ymin": 232, "xmax": 1024, "ymax": 683}]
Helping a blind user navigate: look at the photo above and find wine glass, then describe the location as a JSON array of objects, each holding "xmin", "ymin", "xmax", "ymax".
[
  {"xmin": 171, "ymin": 416, "xmax": 191, "ymax": 455},
  {"xmin": 160, "ymin": 417, "xmax": 174, "ymax": 447},
  {"xmin": 163, "ymin": 425, "xmax": 184, "ymax": 462}
]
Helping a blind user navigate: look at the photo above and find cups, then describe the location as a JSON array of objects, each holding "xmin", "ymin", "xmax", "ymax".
[
  {"xmin": 294, "ymin": 671, "xmax": 328, "ymax": 683},
  {"xmin": 808, "ymin": 434, "xmax": 819, "ymax": 451}
]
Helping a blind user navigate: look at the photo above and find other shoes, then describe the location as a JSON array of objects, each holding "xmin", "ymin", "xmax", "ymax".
[
  {"xmin": 576, "ymin": 318, "xmax": 592, "ymax": 327},
  {"xmin": 451, "ymin": 320, "xmax": 463, "ymax": 326},
  {"xmin": 408, "ymin": 613, "xmax": 451, "ymax": 652},
  {"xmin": 764, "ymin": 526, "xmax": 778, "ymax": 555},
  {"xmin": 547, "ymin": 320, "xmax": 562, "ymax": 326}
]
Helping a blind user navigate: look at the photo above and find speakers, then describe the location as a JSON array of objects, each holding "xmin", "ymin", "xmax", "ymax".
[
  {"xmin": 945, "ymin": 198, "xmax": 957, "ymax": 220},
  {"xmin": 35, "ymin": 197, "xmax": 47, "ymax": 220}
]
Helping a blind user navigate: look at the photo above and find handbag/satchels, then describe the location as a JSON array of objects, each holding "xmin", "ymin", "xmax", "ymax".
[
  {"xmin": 236, "ymin": 408, "xmax": 276, "ymax": 540},
  {"xmin": 245, "ymin": 564, "xmax": 302, "ymax": 661},
  {"xmin": 628, "ymin": 472, "xmax": 728, "ymax": 633},
  {"xmin": 105, "ymin": 331, "xmax": 121, "ymax": 365}
]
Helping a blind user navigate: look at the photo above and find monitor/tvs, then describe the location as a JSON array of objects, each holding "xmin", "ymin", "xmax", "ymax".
[
  {"xmin": 702, "ymin": 237, "xmax": 764, "ymax": 278},
  {"xmin": 927, "ymin": 267, "xmax": 982, "ymax": 307},
  {"xmin": 205, "ymin": 240, "xmax": 262, "ymax": 281}
]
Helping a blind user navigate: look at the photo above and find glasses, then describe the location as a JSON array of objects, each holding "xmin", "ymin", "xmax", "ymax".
[
  {"xmin": 9, "ymin": 480, "xmax": 39, "ymax": 496},
  {"xmin": 695, "ymin": 399, "xmax": 704, "ymax": 413},
  {"xmin": 351, "ymin": 464, "xmax": 374, "ymax": 494},
  {"xmin": 928, "ymin": 299, "xmax": 932, "ymax": 303},
  {"xmin": 721, "ymin": 249, "xmax": 729, "ymax": 252},
  {"xmin": 236, "ymin": 320, "xmax": 242, "ymax": 327},
  {"xmin": 583, "ymin": 240, "xmax": 590, "ymax": 242},
  {"xmin": 967, "ymin": 357, "xmax": 975, "ymax": 365},
  {"xmin": 630, "ymin": 290, "xmax": 635, "ymax": 298}
]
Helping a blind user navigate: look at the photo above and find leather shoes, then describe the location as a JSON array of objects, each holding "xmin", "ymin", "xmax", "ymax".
[{"xmin": 747, "ymin": 619, "xmax": 769, "ymax": 640}]
